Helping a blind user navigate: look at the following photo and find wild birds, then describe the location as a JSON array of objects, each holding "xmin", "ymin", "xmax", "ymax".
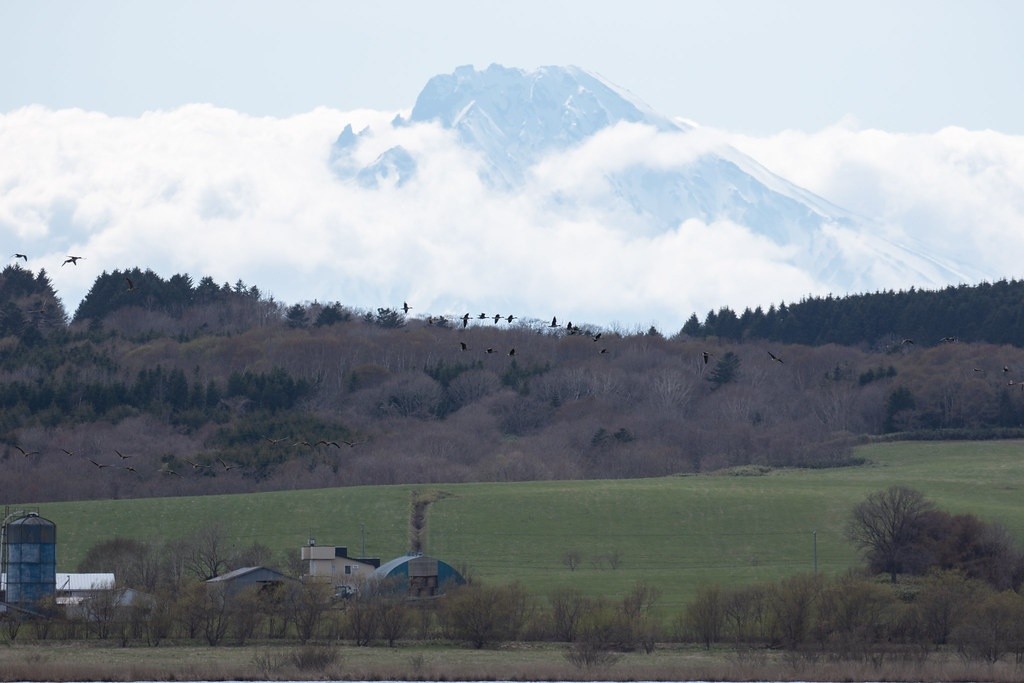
[
  {"xmin": 125, "ymin": 277, "xmax": 140, "ymax": 294},
  {"xmin": 901, "ymin": 339, "xmax": 915, "ymax": 345},
  {"xmin": 62, "ymin": 255, "xmax": 87, "ymax": 266},
  {"xmin": 1006, "ymin": 378, "xmax": 1017, "ymax": 386},
  {"xmin": 401, "ymin": 301, "xmax": 412, "ymax": 314},
  {"xmin": 457, "ymin": 314, "xmax": 609, "ymax": 358},
  {"xmin": 974, "ymin": 367, "xmax": 984, "ymax": 372},
  {"xmin": 1003, "ymin": 363, "xmax": 1010, "ymax": 374},
  {"xmin": 1018, "ymin": 381, "xmax": 1024, "ymax": 391},
  {"xmin": 701, "ymin": 351, "xmax": 713, "ymax": 364},
  {"xmin": 768, "ymin": 351, "xmax": 785, "ymax": 364},
  {"xmin": 10, "ymin": 253, "xmax": 28, "ymax": 262},
  {"xmin": 26, "ymin": 297, "xmax": 48, "ymax": 314},
  {"xmin": 11, "ymin": 435, "xmax": 363, "ymax": 475}
]
